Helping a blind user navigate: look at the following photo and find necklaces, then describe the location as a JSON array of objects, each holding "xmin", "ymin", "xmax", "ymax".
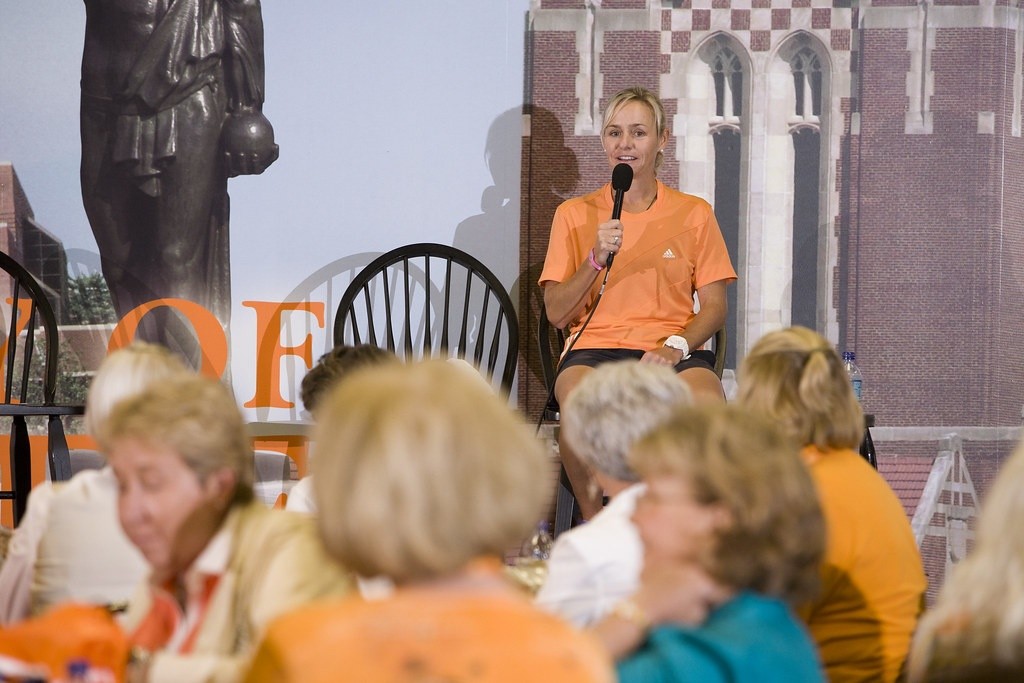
[{"xmin": 646, "ymin": 194, "xmax": 656, "ymax": 210}]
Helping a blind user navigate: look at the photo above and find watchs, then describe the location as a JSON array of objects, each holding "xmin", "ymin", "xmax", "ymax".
[
  {"xmin": 589, "ymin": 247, "xmax": 606, "ymax": 270},
  {"xmin": 662, "ymin": 335, "xmax": 691, "ymax": 360}
]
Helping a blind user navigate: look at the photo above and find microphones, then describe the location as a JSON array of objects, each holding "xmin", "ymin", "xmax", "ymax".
[{"xmin": 606, "ymin": 164, "xmax": 633, "ymax": 268}]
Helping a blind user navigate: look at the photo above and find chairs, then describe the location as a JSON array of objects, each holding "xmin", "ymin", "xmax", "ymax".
[
  {"xmin": 537, "ymin": 297, "xmax": 726, "ymax": 535},
  {"xmin": 334, "ymin": 242, "xmax": 521, "ymax": 410},
  {"xmin": 0, "ymin": 249, "xmax": 87, "ymax": 528}
]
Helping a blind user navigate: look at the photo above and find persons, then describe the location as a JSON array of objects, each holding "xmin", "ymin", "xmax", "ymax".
[
  {"xmin": 537, "ymin": 87, "xmax": 739, "ymax": 527},
  {"xmin": 0, "ymin": 326, "xmax": 1023, "ymax": 683},
  {"xmin": 80, "ymin": 0, "xmax": 280, "ymax": 406}
]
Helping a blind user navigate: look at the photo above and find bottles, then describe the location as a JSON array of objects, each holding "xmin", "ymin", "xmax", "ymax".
[
  {"xmin": 530, "ymin": 521, "xmax": 552, "ymax": 561},
  {"xmin": 859, "ymin": 415, "xmax": 878, "ymax": 472},
  {"xmin": 841, "ymin": 351, "xmax": 864, "ymax": 404}
]
